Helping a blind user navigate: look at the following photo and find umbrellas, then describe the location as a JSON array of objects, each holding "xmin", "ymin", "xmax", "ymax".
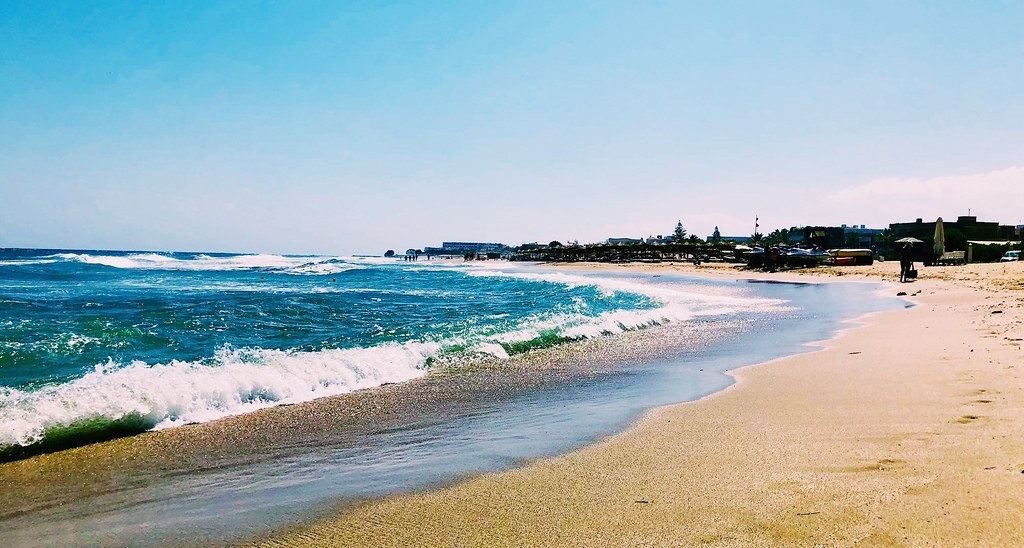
[
  {"xmin": 934, "ymin": 217, "xmax": 945, "ymax": 266},
  {"xmin": 516, "ymin": 240, "xmax": 736, "ymax": 261},
  {"xmin": 894, "ymin": 237, "xmax": 923, "ymax": 242}
]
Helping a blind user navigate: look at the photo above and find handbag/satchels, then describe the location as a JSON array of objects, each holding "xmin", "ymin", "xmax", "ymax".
[{"xmin": 905, "ymin": 264, "xmax": 917, "ymax": 278}]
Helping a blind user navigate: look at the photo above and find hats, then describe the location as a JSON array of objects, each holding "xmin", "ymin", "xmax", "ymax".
[{"xmin": 906, "ymin": 241, "xmax": 913, "ymax": 248}]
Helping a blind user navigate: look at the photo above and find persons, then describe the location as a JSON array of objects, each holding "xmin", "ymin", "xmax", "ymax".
[
  {"xmin": 764, "ymin": 243, "xmax": 778, "ymax": 273},
  {"xmin": 811, "ymin": 243, "xmax": 832, "ymax": 256},
  {"xmin": 899, "ymin": 242, "xmax": 913, "ymax": 282},
  {"xmin": 405, "ymin": 254, "xmax": 453, "ymax": 261},
  {"xmin": 464, "ymin": 252, "xmax": 480, "ymax": 262}
]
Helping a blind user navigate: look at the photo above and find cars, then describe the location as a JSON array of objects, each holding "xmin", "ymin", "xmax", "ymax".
[{"xmin": 1000, "ymin": 250, "xmax": 1021, "ymax": 262}]
